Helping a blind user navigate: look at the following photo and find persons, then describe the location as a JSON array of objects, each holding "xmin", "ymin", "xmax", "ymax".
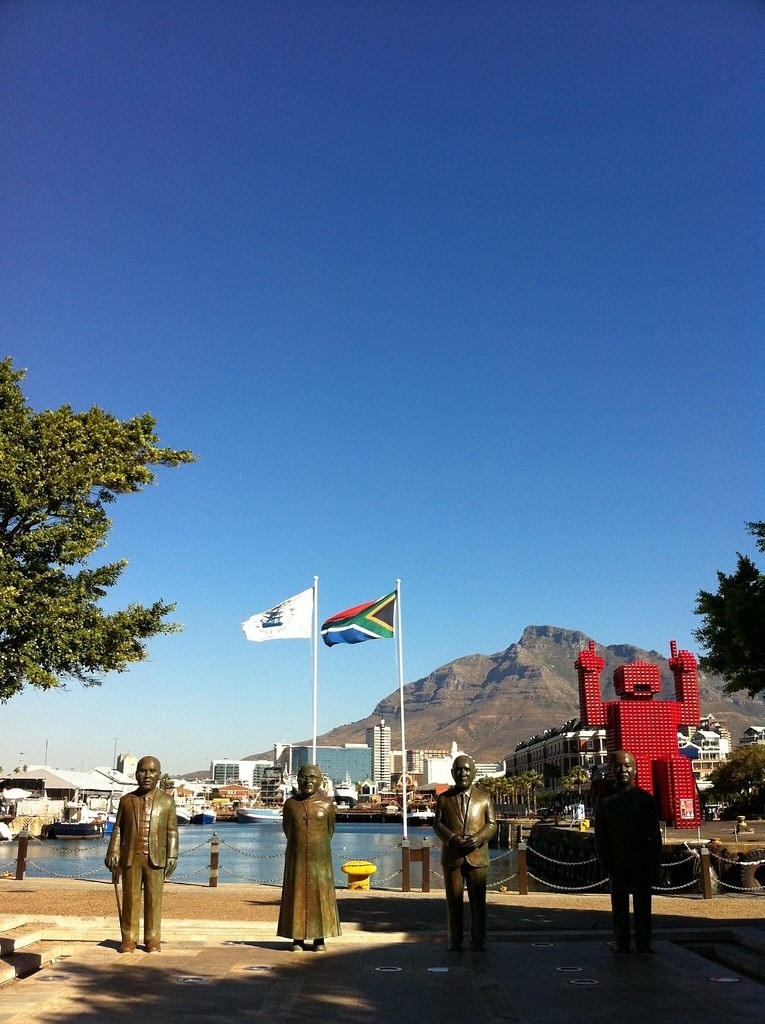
[
  {"xmin": 594, "ymin": 750, "xmax": 663, "ymax": 959},
  {"xmin": 276, "ymin": 764, "xmax": 343, "ymax": 952},
  {"xmin": 432, "ymin": 755, "xmax": 497, "ymax": 953},
  {"xmin": 103, "ymin": 756, "xmax": 179, "ymax": 954}
]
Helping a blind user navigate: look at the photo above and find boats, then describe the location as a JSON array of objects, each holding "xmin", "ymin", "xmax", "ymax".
[
  {"xmin": 103, "ymin": 813, "xmax": 116, "ymax": 833},
  {"xmin": 333, "ymin": 770, "xmax": 358, "ymax": 809},
  {"xmin": 41, "ymin": 802, "xmax": 106, "ymax": 839},
  {"xmin": 383, "ymin": 804, "xmax": 436, "ymax": 826},
  {"xmin": 176, "ymin": 805, "xmax": 218, "ymax": 824},
  {"xmin": 238, "ymin": 808, "xmax": 284, "ymax": 825}
]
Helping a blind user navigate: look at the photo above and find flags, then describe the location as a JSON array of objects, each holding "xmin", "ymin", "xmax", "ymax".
[
  {"xmin": 320, "ymin": 589, "xmax": 398, "ymax": 648},
  {"xmin": 240, "ymin": 587, "xmax": 314, "ymax": 643}
]
